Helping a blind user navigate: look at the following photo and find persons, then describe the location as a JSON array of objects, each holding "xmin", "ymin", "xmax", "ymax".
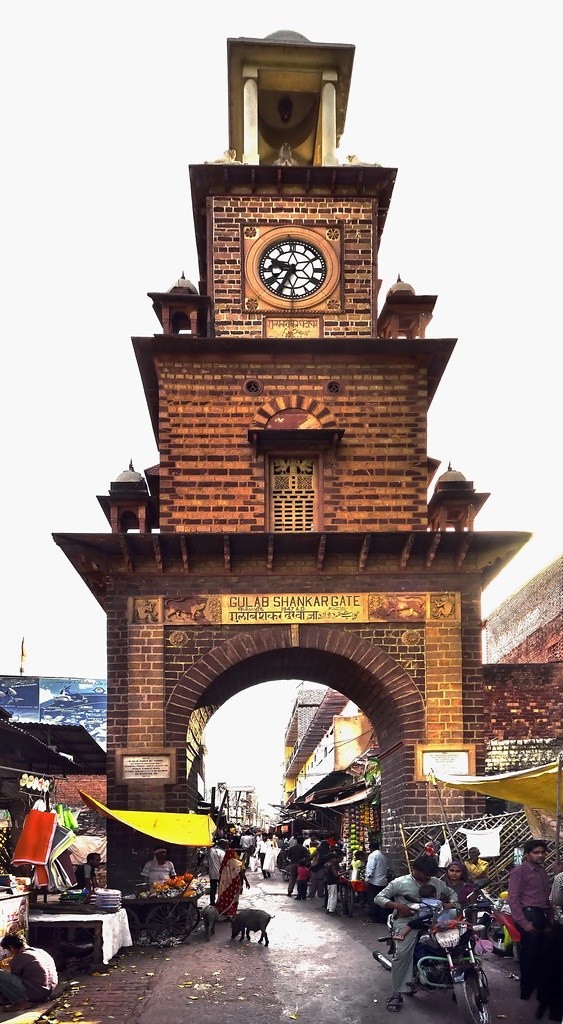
[
  {"xmin": 374, "ymin": 856, "xmax": 458, "ymax": 1013},
  {"xmin": 75, "ymin": 853, "xmax": 106, "ymax": 888},
  {"xmin": 364, "ymin": 843, "xmax": 388, "ymax": 924},
  {"xmin": 277, "ymin": 832, "xmax": 345, "ymax": 915},
  {"xmin": 209, "ymin": 839, "xmax": 250, "ymax": 919},
  {"xmin": 0, "ymin": 932, "xmax": 58, "ymax": 1011},
  {"xmin": 445, "ymin": 861, "xmax": 473, "ymax": 898},
  {"xmin": 350, "ymin": 851, "xmax": 366, "ymax": 870},
  {"xmin": 140, "ymin": 846, "xmax": 177, "ymax": 884},
  {"xmin": 465, "ymin": 847, "xmax": 489, "ymax": 894},
  {"xmin": 509, "ymin": 839, "xmax": 554, "ymax": 1001},
  {"xmin": 238, "ymin": 830, "xmax": 276, "ymax": 879}
]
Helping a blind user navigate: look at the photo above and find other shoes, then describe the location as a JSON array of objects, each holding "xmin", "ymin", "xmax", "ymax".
[
  {"xmin": 294, "ymin": 896, "xmax": 301, "ymax": 900},
  {"xmin": 327, "ymin": 909, "xmax": 340, "ymax": 916},
  {"xmin": 288, "ymin": 893, "xmax": 291, "ymax": 897},
  {"xmin": 393, "ymin": 934, "xmax": 404, "ymax": 940},
  {"xmin": 302, "ymin": 897, "xmax": 306, "ymax": 899}
]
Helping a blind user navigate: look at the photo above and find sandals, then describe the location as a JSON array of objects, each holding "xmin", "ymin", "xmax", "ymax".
[{"xmin": 387, "ymin": 993, "xmax": 403, "ymax": 1011}]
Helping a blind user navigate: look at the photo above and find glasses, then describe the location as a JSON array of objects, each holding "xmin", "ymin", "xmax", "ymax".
[{"xmin": 448, "ymin": 869, "xmax": 463, "ymax": 872}]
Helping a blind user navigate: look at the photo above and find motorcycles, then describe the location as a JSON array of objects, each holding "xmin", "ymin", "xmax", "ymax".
[{"xmin": 373, "ymin": 878, "xmax": 495, "ymax": 1024}]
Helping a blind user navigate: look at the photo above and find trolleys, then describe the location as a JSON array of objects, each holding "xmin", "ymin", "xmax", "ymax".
[
  {"xmin": 121, "ymin": 882, "xmax": 206, "ymax": 947},
  {"xmin": 338, "ymin": 871, "xmax": 368, "ymax": 918}
]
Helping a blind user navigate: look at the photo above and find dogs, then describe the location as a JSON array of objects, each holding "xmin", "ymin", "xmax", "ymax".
[
  {"xmin": 201, "ymin": 905, "xmax": 220, "ymax": 941},
  {"xmin": 228, "ymin": 908, "xmax": 275, "ymax": 947}
]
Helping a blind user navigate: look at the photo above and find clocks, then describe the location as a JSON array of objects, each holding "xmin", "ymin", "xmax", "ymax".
[{"xmin": 257, "ymin": 239, "xmax": 327, "ymax": 299}]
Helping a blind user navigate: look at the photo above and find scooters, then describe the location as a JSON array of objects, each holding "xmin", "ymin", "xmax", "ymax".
[{"xmin": 279, "ymin": 857, "xmax": 313, "ymax": 886}]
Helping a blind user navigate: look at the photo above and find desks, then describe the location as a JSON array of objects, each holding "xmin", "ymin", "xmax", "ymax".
[
  {"xmin": 28, "ymin": 909, "xmax": 132, "ymax": 973},
  {"xmin": 339, "ymin": 880, "xmax": 371, "ymax": 918}
]
